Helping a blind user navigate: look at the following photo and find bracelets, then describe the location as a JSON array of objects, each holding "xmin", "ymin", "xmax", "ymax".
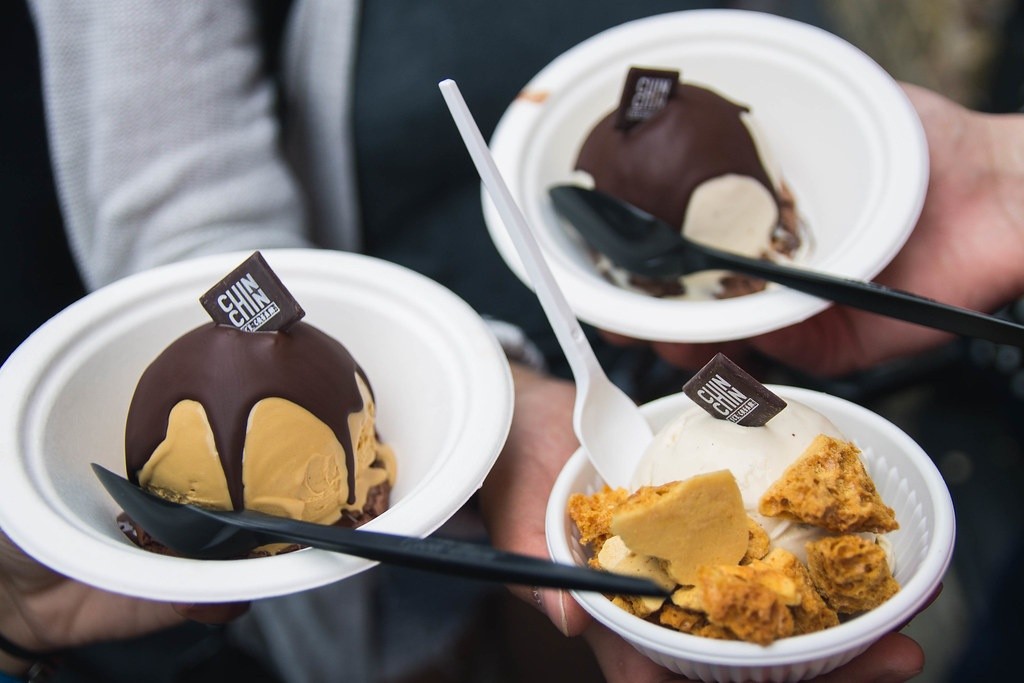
[{"xmin": 0, "ymin": 635, "xmax": 46, "ymax": 664}]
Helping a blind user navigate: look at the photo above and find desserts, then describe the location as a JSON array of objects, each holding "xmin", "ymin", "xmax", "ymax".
[
  {"xmin": 536, "ymin": 64, "xmax": 815, "ymax": 303},
  {"xmin": 111, "ymin": 250, "xmax": 400, "ymax": 561},
  {"xmin": 566, "ymin": 352, "xmax": 902, "ymax": 649}
]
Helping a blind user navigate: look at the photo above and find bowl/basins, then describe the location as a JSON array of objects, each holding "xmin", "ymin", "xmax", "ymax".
[
  {"xmin": 0, "ymin": 248, "xmax": 517, "ymax": 603},
  {"xmin": 543, "ymin": 381, "xmax": 955, "ymax": 683},
  {"xmin": 478, "ymin": 8, "xmax": 930, "ymax": 343}
]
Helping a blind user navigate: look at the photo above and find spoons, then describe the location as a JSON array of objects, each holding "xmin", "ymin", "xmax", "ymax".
[
  {"xmin": 90, "ymin": 461, "xmax": 669, "ymax": 599},
  {"xmin": 545, "ymin": 185, "xmax": 1023, "ymax": 348},
  {"xmin": 436, "ymin": 80, "xmax": 653, "ymax": 493}
]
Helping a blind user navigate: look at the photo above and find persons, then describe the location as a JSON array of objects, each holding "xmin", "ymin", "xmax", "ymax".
[
  {"xmin": 599, "ymin": 75, "xmax": 1024, "ymax": 373},
  {"xmin": 26, "ymin": 0, "xmax": 942, "ymax": 683},
  {"xmin": 0, "ymin": 531, "xmax": 249, "ymax": 683}
]
